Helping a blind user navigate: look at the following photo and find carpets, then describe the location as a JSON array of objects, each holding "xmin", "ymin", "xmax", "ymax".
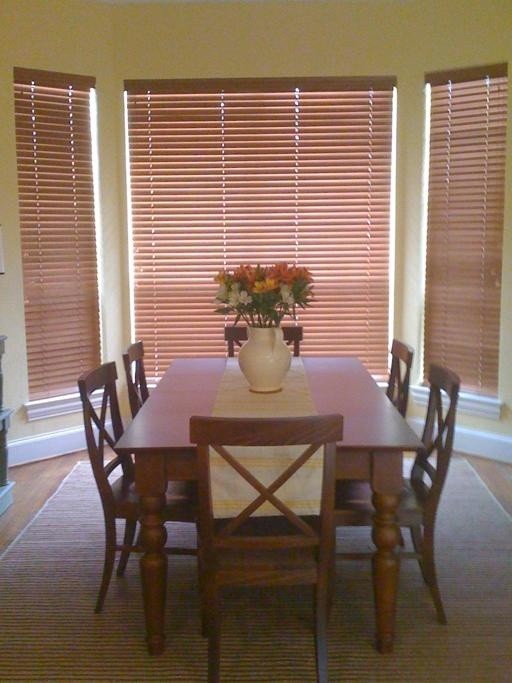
[{"xmin": 0, "ymin": 458, "xmax": 512, "ymax": 683}]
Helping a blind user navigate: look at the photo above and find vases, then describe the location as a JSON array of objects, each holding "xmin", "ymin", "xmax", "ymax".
[{"xmin": 239, "ymin": 328, "xmax": 291, "ymax": 393}]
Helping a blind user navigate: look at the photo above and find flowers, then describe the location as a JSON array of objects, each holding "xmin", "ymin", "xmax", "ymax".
[{"xmin": 211, "ymin": 262, "xmax": 314, "ymax": 336}]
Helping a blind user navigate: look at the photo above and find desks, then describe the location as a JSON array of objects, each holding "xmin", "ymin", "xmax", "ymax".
[{"xmin": 113, "ymin": 358, "xmax": 424, "ymax": 655}]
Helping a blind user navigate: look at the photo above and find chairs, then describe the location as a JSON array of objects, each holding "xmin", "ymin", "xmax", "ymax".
[
  {"xmin": 225, "ymin": 327, "xmax": 303, "ymax": 357},
  {"xmin": 325, "ymin": 363, "xmax": 461, "ymax": 624},
  {"xmin": 77, "ymin": 361, "xmax": 211, "ymax": 614},
  {"xmin": 386, "ymin": 339, "xmax": 413, "ymax": 417},
  {"xmin": 190, "ymin": 415, "xmax": 344, "ymax": 683},
  {"xmin": 123, "ymin": 341, "xmax": 150, "ymax": 420}
]
